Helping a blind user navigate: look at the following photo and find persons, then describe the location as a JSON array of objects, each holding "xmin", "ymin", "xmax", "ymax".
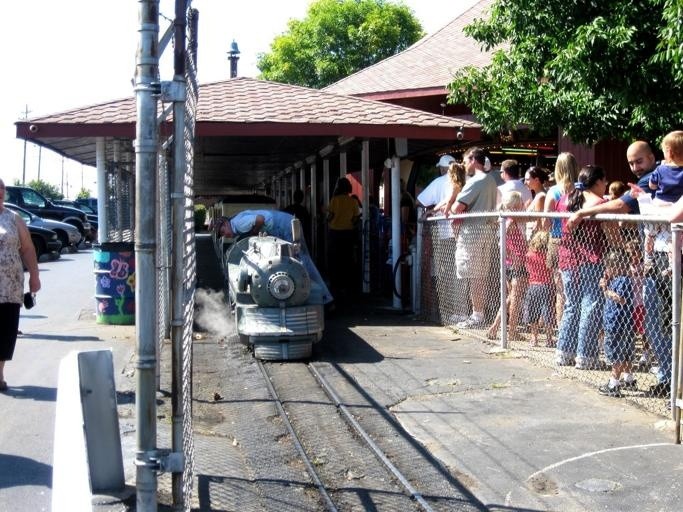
[
  {"xmin": 555, "ymin": 166, "xmax": 620, "ymax": 370},
  {"xmin": 644, "ymin": 134, "xmax": 683, "ymax": 282},
  {"xmin": 370, "ymin": 195, "xmax": 385, "ymax": 241},
  {"xmin": 415, "ymin": 155, "xmax": 468, "ymax": 322},
  {"xmin": 567, "ymin": 141, "xmax": 672, "ymax": 398},
  {"xmin": 628, "ymin": 182, "xmax": 683, "ymax": 333},
  {"xmin": 326, "ymin": 177, "xmax": 361, "ymax": 295},
  {"xmin": 398, "ymin": 179, "xmax": 416, "ymax": 249},
  {"xmin": 286, "ymin": 190, "xmax": 311, "ymax": 237},
  {"xmin": 0, "ymin": 180, "xmax": 40, "ymax": 392},
  {"xmin": 483, "ymin": 152, "xmax": 580, "ymax": 347},
  {"xmin": 440, "ymin": 147, "xmax": 497, "ymax": 329},
  {"xmin": 444, "ymin": 164, "xmax": 466, "ymax": 228},
  {"xmin": 215, "ymin": 210, "xmax": 334, "ymax": 307},
  {"xmin": 598, "ymin": 182, "xmax": 653, "ymax": 396}
]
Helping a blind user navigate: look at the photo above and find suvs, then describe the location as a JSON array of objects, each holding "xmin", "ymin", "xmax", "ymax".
[{"xmin": 0, "ymin": 185, "xmax": 100, "ymax": 261}]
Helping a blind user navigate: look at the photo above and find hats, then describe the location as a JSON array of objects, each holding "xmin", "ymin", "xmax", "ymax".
[
  {"xmin": 435, "ymin": 155, "xmax": 456, "ymax": 168},
  {"xmin": 214, "ymin": 216, "xmax": 228, "ymax": 239}
]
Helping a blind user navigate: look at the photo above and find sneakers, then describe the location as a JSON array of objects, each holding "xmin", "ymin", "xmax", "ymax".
[
  {"xmin": 0, "ymin": 380, "xmax": 7, "ymax": 391},
  {"xmin": 619, "ymin": 377, "xmax": 638, "ymax": 391},
  {"xmin": 650, "ymin": 381, "xmax": 671, "ymax": 398},
  {"xmin": 597, "ymin": 379, "xmax": 621, "ymax": 397},
  {"xmin": 575, "ymin": 355, "xmax": 607, "ymax": 370},
  {"xmin": 639, "ymin": 351, "xmax": 652, "ymax": 365},
  {"xmin": 555, "ymin": 355, "xmax": 575, "ymax": 366}
]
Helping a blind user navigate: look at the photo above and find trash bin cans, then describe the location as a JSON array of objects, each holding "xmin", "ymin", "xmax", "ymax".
[{"xmin": 91, "ymin": 242, "xmax": 135, "ymax": 325}]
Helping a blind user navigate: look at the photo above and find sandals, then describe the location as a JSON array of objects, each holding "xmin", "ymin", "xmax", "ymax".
[{"xmin": 447, "ymin": 310, "xmax": 557, "ymax": 347}]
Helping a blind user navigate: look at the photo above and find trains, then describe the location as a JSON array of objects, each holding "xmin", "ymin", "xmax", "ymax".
[{"xmin": 206, "ymin": 193, "xmax": 329, "ymax": 365}]
